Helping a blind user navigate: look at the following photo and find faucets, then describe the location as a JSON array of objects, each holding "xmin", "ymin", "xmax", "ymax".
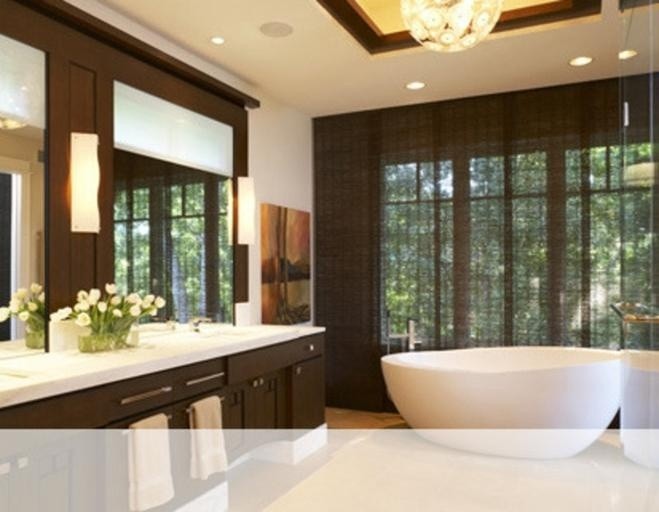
[
  {"xmin": 407, "ymin": 319, "xmax": 422, "ymax": 351},
  {"xmin": 152, "ymin": 317, "xmax": 170, "ymax": 324},
  {"xmin": 193, "ymin": 318, "xmax": 211, "ymax": 327}
]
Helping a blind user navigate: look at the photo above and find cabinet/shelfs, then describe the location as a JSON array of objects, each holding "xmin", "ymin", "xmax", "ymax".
[{"xmin": 1, "ymin": 332, "xmax": 331, "ymax": 512}]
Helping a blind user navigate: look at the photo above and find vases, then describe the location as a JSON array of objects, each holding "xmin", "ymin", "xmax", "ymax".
[
  {"xmin": 25, "ymin": 325, "xmax": 46, "ymax": 349},
  {"xmin": 78, "ymin": 314, "xmax": 139, "ymax": 352}
]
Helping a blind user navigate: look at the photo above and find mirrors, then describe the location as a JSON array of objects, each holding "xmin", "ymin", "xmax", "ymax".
[
  {"xmin": 114, "ymin": 80, "xmax": 238, "ymax": 337},
  {"xmin": 0, "ymin": 32, "xmax": 48, "ymax": 360}
]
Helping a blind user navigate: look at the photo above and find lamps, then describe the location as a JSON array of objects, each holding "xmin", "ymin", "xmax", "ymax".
[{"xmin": 400, "ymin": 0, "xmax": 502, "ymax": 54}]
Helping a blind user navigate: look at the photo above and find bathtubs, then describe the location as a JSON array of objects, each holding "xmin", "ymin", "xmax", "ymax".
[{"xmin": 380, "ymin": 347, "xmax": 629, "ymax": 462}]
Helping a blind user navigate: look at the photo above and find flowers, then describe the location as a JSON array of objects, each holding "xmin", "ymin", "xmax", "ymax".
[
  {"xmin": 48, "ymin": 280, "xmax": 167, "ymax": 352},
  {"xmin": 7, "ymin": 280, "xmax": 45, "ymax": 332}
]
